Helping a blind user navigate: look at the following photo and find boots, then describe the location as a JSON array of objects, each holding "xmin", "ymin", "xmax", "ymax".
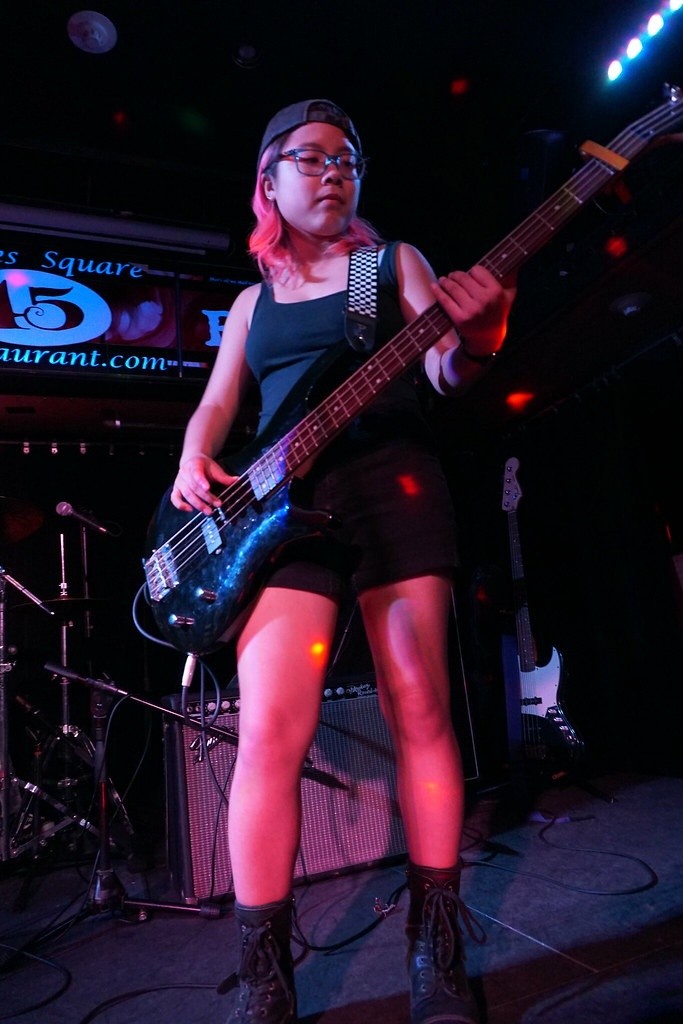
[
  {"xmin": 216, "ymin": 893, "xmax": 298, "ymax": 1024},
  {"xmin": 405, "ymin": 857, "xmax": 488, "ymax": 1024}
]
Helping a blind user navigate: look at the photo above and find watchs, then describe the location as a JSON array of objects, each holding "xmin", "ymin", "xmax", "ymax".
[{"xmin": 460, "ymin": 338, "xmax": 514, "ymax": 380}]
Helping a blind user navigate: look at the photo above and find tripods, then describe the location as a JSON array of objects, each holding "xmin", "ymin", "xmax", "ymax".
[{"xmin": 0, "ymin": 525, "xmax": 352, "ymax": 971}]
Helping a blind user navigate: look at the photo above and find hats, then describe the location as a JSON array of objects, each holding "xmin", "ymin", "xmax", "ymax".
[{"xmin": 257, "ymin": 100, "xmax": 362, "ymax": 153}]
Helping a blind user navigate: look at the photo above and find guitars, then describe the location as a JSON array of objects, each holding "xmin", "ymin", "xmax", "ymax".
[
  {"xmin": 501, "ymin": 455, "xmax": 586, "ymax": 765},
  {"xmin": 133, "ymin": 84, "xmax": 683, "ymax": 652}
]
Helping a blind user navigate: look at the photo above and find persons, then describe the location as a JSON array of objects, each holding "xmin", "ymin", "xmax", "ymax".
[{"xmin": 168, "ymin": 100, "xmax": 517, "ymax": 1023}]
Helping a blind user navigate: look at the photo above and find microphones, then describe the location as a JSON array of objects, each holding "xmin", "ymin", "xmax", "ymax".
[{"xmin": 56, "ymin": 503, "xmax": 111, "ymax": 534}]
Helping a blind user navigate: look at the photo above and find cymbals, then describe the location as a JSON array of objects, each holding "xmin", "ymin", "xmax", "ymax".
[{"xmin": 9, "ymin": 595, "xmax": 108, "ymax": 620}]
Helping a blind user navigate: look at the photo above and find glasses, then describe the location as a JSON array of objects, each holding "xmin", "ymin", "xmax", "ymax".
[{"xmin": 265, "ymin": 149, "xmax": 365, "ymax": 180}]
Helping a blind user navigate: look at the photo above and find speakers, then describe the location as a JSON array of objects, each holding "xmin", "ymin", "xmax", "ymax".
[{"xmin": 157, "ymin": 676, "xmax": 411, "ymax": 907}]
